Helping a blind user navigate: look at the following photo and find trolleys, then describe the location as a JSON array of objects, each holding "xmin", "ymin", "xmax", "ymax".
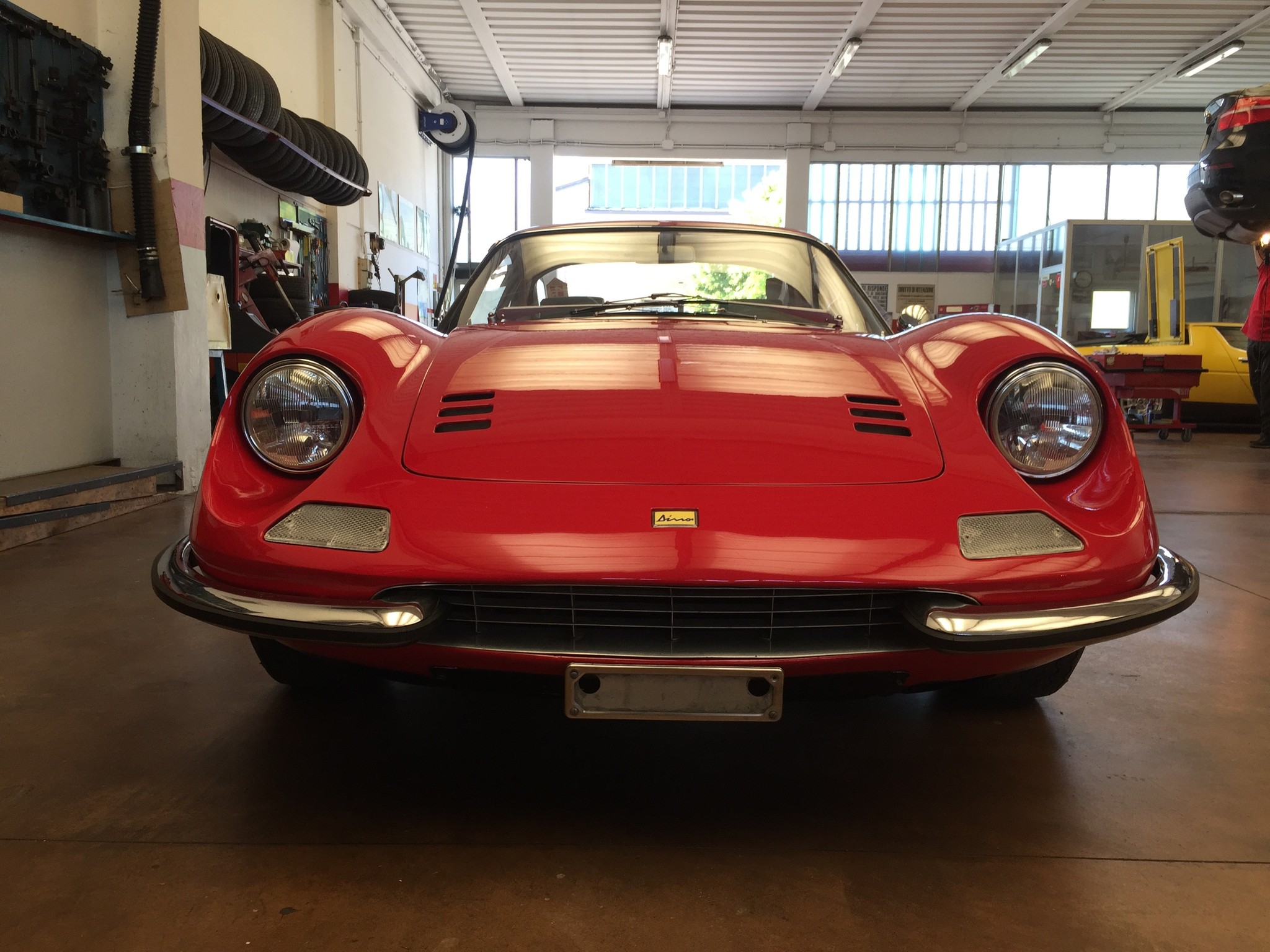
[{"xmin": 1082, "ymin": 353, "xmax": 1209, "ymax": 442}]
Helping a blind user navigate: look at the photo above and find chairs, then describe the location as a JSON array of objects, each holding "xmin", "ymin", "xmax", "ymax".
[
  {"xmin": 720, "ymin": 299, "xmax": 784, "ymax": 322},
  {"xmin": 541, "ymin": 297, "xmax": 604, "ymax": 320}
]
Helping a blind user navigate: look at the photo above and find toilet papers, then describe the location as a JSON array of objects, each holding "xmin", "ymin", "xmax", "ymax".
[{"xmin": 280, "ymin": 238, "xmax": 300, "ymax": 263}]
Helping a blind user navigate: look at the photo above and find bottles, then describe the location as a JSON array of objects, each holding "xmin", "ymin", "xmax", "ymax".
[
  {"xmin": 1148, "ymin": 410, "xmax": 1152, "ymax": 424},
  {"xmin": 1146, "ymin": 399, "xmax": 1154, "ymax": 419}
]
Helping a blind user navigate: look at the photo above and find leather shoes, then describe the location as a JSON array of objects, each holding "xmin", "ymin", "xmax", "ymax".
[{"xmin": 1249, "ymin": 433, "xmax": 1270, "ymax": 448}]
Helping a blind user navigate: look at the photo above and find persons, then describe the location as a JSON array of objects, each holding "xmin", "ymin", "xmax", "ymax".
[{"xmin": 1239, "ymin": 239, "xmax": 1270, "ymax": 449}]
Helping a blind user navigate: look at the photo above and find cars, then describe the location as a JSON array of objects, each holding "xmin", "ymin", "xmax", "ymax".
[{"xmin": 1183, "ymin": 82, "xmax": 1270, "ymax": 247}]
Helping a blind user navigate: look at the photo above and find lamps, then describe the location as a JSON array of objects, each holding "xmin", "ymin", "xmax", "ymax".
[
  {"xmin": 1175, "ymin": 40, "xmax": 1245, "ymax": 77},
  {"xmin": 656, "ymin": 35, "xmax": 673, "ymax": 76},
  {"xmin": 1001, "ymin": 38, "xmax": 1052, "ymax": 79},
  {"xmin": 829, "ymin": 37, "xmax": 863, "ymax": 77}
]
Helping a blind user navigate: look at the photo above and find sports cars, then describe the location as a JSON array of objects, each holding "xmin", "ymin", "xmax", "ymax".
[{"xmin": 149, "ymin": 218, "xmax": 1201, "ymax": 723}]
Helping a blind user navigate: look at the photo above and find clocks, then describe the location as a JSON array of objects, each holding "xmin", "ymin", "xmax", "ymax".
[{"xmin": 1074, "ymin": 270, "xmax": 1092, "ymax": 288}]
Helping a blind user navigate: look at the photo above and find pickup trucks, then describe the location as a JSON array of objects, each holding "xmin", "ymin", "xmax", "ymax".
[{"xmin": 1070, "ymin": 236, "xmax": 1261, "ymax": 419}]
